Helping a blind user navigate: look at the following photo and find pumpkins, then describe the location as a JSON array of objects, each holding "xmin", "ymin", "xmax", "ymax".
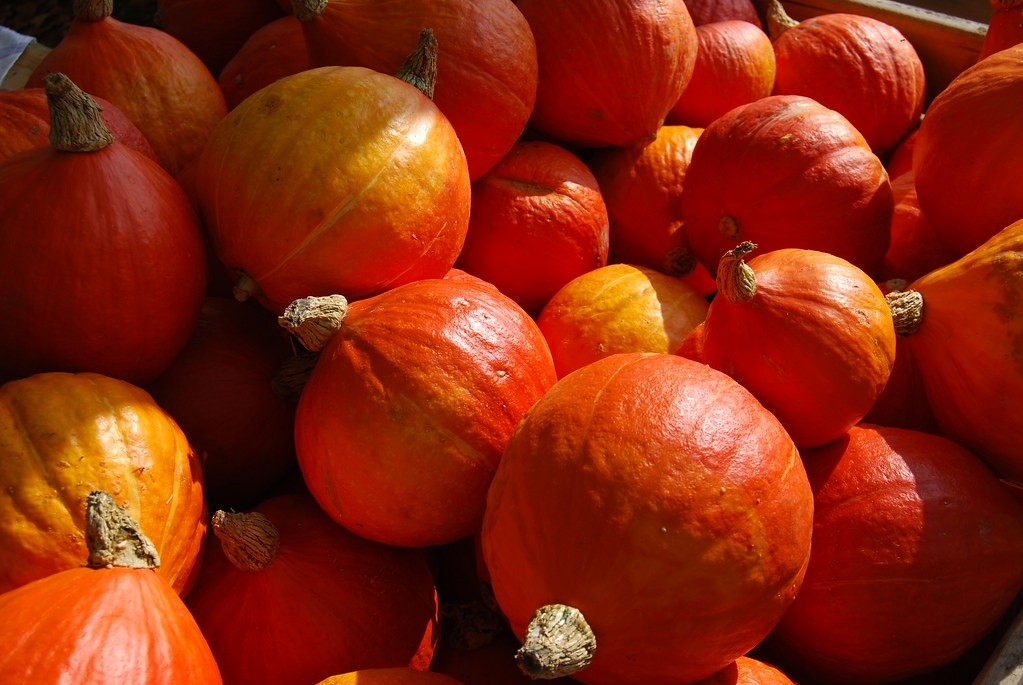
[{"xmin": 0, "ymin": 0, "xmax": 1023, "ymax": 685}]
